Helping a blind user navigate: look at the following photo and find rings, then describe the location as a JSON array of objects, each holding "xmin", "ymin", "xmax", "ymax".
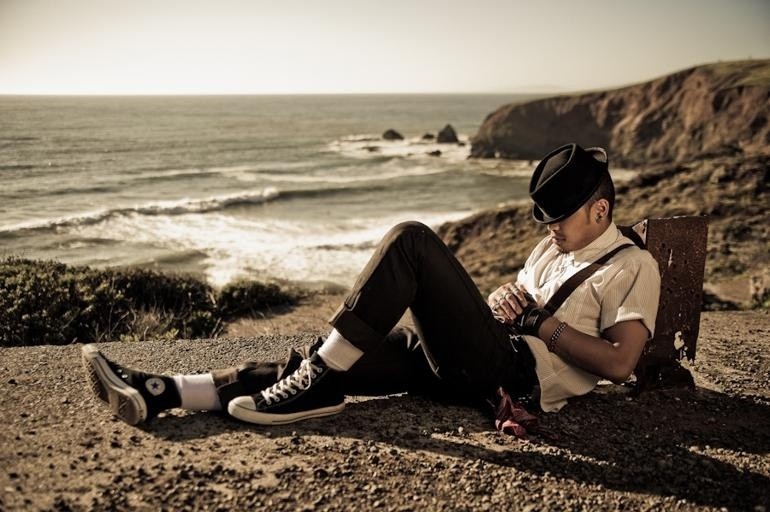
[
  {"xmin": 513, "ymin": 288, "xmax": 519, "ymax": 293},
  {"xmin": 498, "ymin": 297, "xmax": 503, "ymax": 303},
  {"xmin": 492, "ymin": 304, "xmax": 501, "ymax": 311},
  {"xmin": 503, "ymin": 291, "xmax": 513, "ymax": 299}
]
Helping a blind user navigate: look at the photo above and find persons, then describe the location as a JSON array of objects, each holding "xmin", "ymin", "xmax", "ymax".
[{"xmin": 80, "ymin": 142, "xmax": 664, "ymax": 427}]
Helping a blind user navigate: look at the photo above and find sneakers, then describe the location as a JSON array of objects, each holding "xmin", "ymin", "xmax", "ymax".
[
  {"xmin": 81, "ymin": 343, "xmax": 182, "ymax": 425},
  {"xmin": 228, "ymin": 350, "xmax": 347, "ymax": 426}
]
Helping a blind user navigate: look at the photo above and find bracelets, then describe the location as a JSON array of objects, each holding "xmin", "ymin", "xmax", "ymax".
[{"xmin": 547, "ymin": 322, "xmax": 567, "ymax": 352}]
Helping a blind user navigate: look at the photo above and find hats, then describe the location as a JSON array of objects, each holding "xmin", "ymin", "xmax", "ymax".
[{"xmin": 527, "ymin": 141, "xmax": 609, "ymax": 225}]
